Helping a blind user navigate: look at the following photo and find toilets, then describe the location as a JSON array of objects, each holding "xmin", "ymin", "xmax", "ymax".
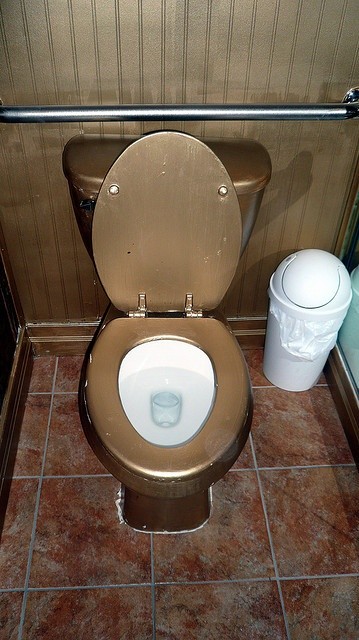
[{"xmin": 61, "ymin": 129, "xmax": 271, "ymax": 532}]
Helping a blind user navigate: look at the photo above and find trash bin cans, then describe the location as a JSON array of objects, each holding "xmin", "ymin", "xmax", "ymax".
[
  {"xmin": 338, "ymin": 265, "xmax": 359, "ymax": 391},
  {"xmin": 262, "ymin": 249, "xmax": 353, "ymax": 393}
]
